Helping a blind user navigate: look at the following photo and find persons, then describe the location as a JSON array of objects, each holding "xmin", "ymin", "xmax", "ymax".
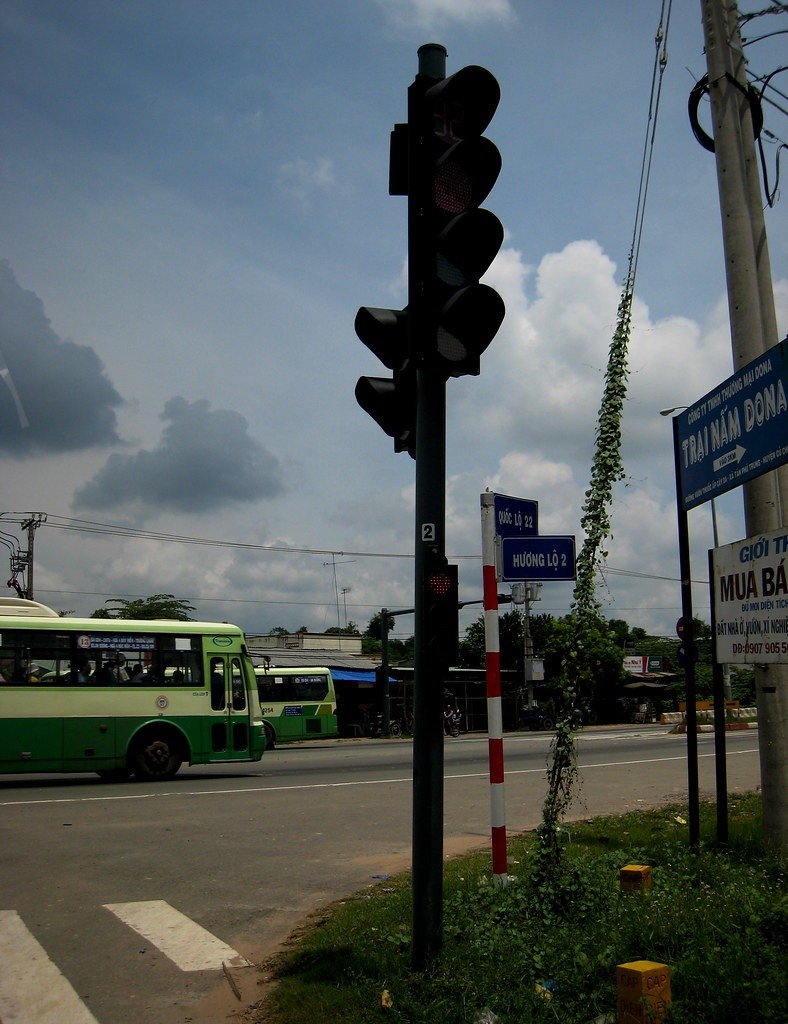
[
  {"xmin": 0, "ymin": 650, "xmax": 225, "ymax": 750},
  {"xmin": 639, "ymin": 700, "xmax": 647, "ymax": 723},
  {"xmin": 444, "ymin": 704, "xmax": 461, "ymax": 735}
]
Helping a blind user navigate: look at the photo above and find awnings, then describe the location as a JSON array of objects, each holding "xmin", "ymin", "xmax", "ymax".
[{"xmin": 330, "ymin": 669, "xmax": 396, "ymax": 684}]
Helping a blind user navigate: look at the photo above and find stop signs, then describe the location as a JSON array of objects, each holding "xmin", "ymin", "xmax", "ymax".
[{"xmin": 675, "ymin": 617, "xmax": 698, "ymax": 643}]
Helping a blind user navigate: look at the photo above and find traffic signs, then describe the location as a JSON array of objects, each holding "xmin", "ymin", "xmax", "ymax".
[{"xmin": 680, "ymin": 337, "xmax": 788, "ymax": 514}]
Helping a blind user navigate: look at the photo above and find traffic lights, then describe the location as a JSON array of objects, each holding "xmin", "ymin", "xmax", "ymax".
[
  {"xmin": 417, "ymin": 563, "xmax": 460, "ymax": 669},
  {"xmin": 354, "ymin": 300, "xmax": 414, "ymax": 457},
  {"xmin": 409, "ymin": 65, "xmax": 505, "ymax": 385}
]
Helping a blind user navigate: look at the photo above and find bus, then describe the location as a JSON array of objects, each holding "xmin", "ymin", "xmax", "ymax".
[
  {"xmin": 40, "ymin": 667, "xmax": 339, "ymax": 751},
  {"xmin": 0, "ymin": 596, "xmax": 267, "ymax": 781}
]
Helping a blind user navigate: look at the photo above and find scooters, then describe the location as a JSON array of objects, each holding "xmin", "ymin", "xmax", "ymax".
[{"xmin": 440, "ymin": 716, "xmax": 460, "ymax": 738}]
